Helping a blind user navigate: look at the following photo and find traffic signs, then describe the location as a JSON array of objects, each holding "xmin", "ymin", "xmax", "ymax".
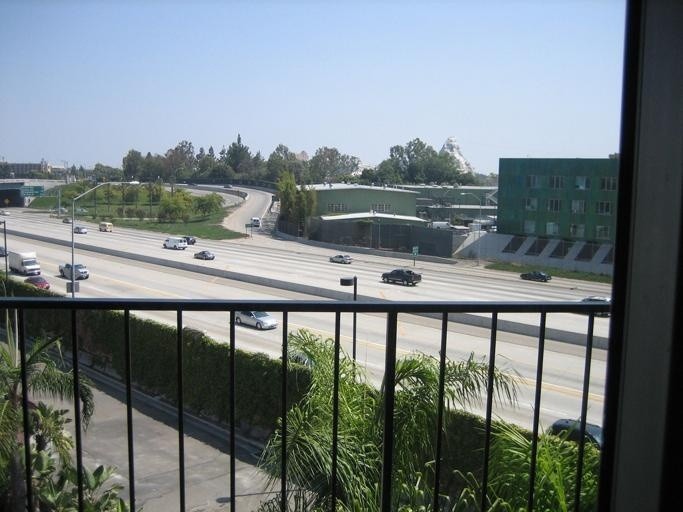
[{"xmin": 21, "ymin": 185, "xmax": 44, "ymax": 198}]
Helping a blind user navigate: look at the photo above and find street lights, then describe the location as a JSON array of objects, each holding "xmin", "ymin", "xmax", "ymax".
[{"xmin": 71, "ymin": 180, "xmax": 140, "ymax": 297}]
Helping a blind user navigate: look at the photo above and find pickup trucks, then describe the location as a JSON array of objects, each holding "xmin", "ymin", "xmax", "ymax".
[{"xmin": 382, "ymin": 269, "xmax": 421, "ymax": 286}]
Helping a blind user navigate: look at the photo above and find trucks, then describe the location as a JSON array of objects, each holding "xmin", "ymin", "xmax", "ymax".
[
  {"xmin": 9, "ymin": 250, "xmax": 40, "ymax": 276},
  {"xmin": 163, "ymin": 237, "xmax": 188, "ymax": 250}
]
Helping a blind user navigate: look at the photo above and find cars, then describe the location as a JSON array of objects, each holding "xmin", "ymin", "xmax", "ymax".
[
  {"xmin": 580, "ymin": 295, "xmax": 612, "ymax": 318},
  {"xmin": 279, "ymin": 352, "xmax": 313, "ymax": 367},
  {"xmin": 330, "ymin": 254, "xmax": 352, "ymax": 265},
  {"xmin": 1, "ymin": 209, "xmax": 9, "ymax": 216},
  {"xmin": 550, "ymin": 418, "xmax": 601, "ymax": 449},
  {"xmin": 74, "ymin": 226, "xmax": 87, "ymax": 234},
  {"xmin": 63, "ymin": 218, "xmax": 72, "ymax": 223},
  {"xmin": 0, "ymin": 247, "xmax": 8, "ymax": 257},
  {"xmin": 224, "ymin": 184, "xmax": 233, "ymax": 188},
  {"xmin": 194, "ymin": 251, "xmax": 215, "ymax": 261},
  {"xmin": 520, "ymin": 271, "xmax": 551, "ymax": 281},
  {"xmin": 235, "ymin": 310, "xmax": 278, "ymax": 330},
  {"xmin": 59, "ymin": 262, "xmax": 89, "ymax": 279},
  {"xmin": 25, "ymin": 276, "xmax": 49, "ymax": 289}
]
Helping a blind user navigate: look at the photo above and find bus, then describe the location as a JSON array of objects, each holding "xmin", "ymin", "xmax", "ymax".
[{"xmin": 99, "ymin": 222, "xmax": 113, "ymax": 232}]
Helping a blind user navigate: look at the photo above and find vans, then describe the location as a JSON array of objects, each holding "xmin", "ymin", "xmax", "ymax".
[{"xmin": 251, "ymin": 218, "xmax": 260, "ymax": 227}]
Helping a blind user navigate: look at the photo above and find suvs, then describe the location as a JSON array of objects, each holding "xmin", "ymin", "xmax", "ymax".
[{"xmin": 184, "ymin": 237, "xmax": 195, "ymax": 245}]
